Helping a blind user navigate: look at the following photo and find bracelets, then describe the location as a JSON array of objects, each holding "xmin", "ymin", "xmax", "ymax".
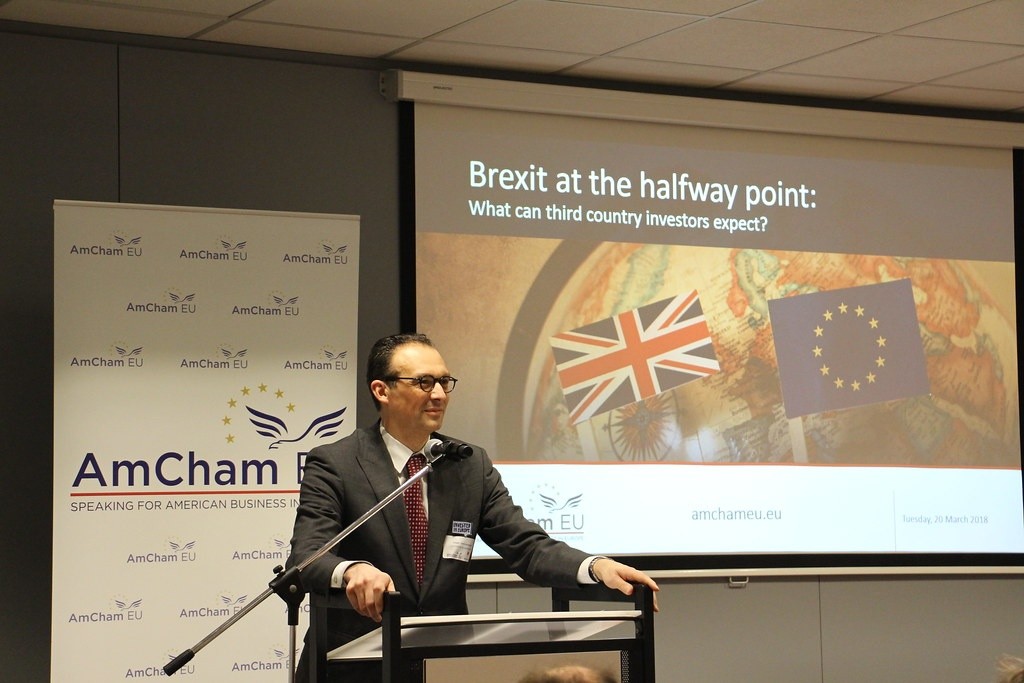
[{"xmin": 589, "ymin": 557, "xmax": 599, "ymax": 582}]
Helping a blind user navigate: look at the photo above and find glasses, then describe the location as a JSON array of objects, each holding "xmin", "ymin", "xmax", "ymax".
[{"xmin": 369, "ymin": 375, "xmax": 458, "ymax": 393}]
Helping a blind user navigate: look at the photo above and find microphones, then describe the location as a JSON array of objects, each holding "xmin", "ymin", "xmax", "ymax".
[{"xmin": 423, "ymin": 437, "xmax": 472, "ymax": 462}]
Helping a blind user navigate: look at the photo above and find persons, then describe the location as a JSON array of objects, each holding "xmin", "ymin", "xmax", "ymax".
[{"xmin": 284, "ymin": 334, "xmax": 659, "ymax": 673}]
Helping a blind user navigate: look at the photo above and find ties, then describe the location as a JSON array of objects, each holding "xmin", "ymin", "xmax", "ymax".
[{"xmin": 403, "ymin": 453, "xmax": 429, "ymax": 592}]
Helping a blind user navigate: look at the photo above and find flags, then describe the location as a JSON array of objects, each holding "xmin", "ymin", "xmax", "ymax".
[
  {"xmin": 549, "ymin": 289, "xmax": 721, "ymax": 426},
  {"xmin": 766, "ymin": 279, "xmax": 931, "ymax": 417}
]
